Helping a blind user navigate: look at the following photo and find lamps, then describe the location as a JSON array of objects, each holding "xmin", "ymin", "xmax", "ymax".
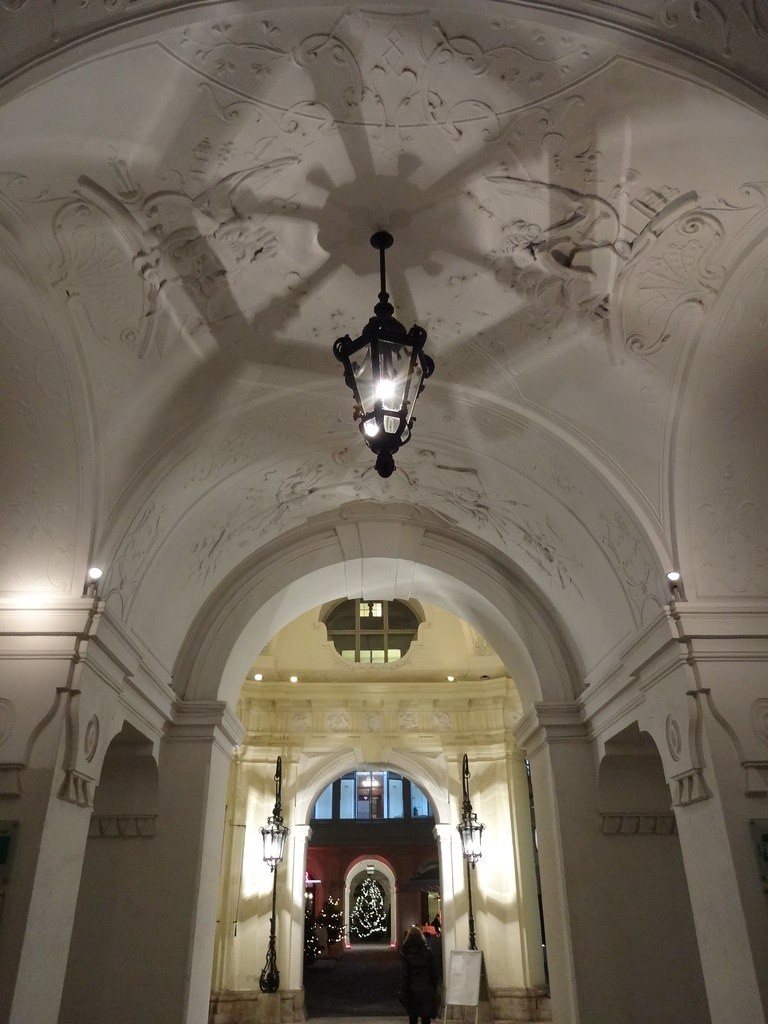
[
  {"xmin": 331, "ymin": 231, "xmax": 435, "ymax": 479},
  {"xmin": 456, "ymin": 754, "xmax": 485, "ymax": 950},
  {"xmin": 259, "ymin": 756, "xmax": 292, "ymax": 993}
]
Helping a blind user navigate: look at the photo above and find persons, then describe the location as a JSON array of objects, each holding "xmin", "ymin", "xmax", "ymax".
[{"xmin": 396, "ymin": 927, "xmax": 441, "ymax": 1024}]
